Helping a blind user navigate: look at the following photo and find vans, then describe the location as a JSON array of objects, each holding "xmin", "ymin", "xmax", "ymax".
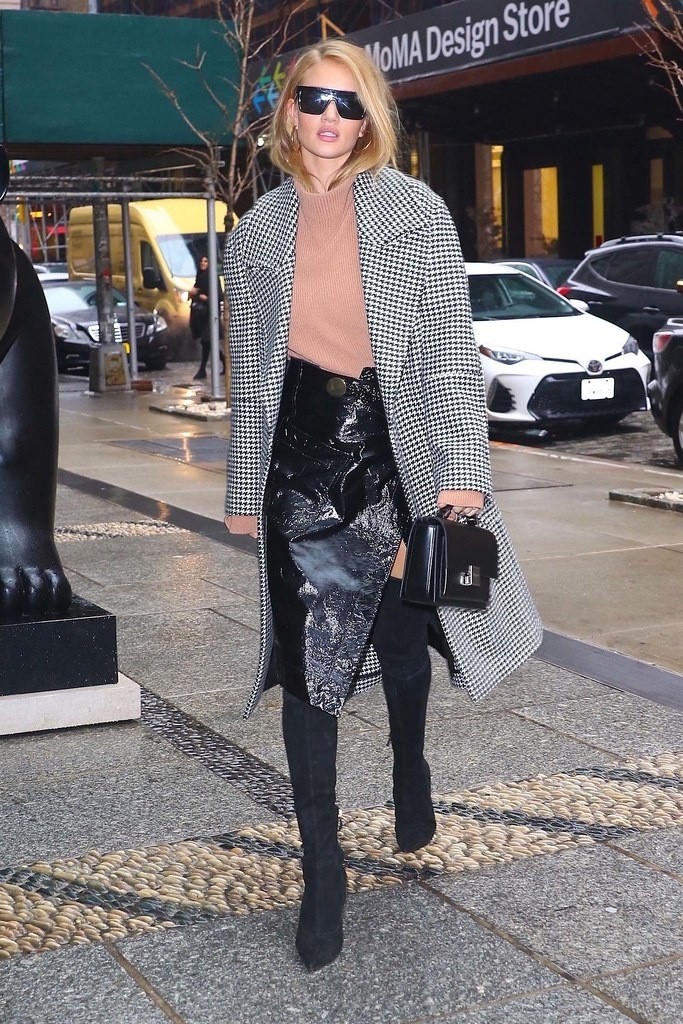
[{"xmin": 67, "ymin": 198, "xmax": 238, "ymax": 342}]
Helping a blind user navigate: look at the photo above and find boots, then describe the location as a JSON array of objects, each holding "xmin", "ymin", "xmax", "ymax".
[
  {"xmin": 372, "ymin": 576, "xmax": 435, "ymax": 854},
  {"xmin": 274, "ymin": 639, "xmax": 348, "ymax": 973}
]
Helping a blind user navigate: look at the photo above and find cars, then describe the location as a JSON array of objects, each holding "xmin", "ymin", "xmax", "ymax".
[
  {"xmin": 32, "ymin": 262, "xmax": 69, "ymax": 281},
  {"xmin": 33, "ymin": 281, "xmax": 170, "ymax": 373},
  {"xmin": 463, "ymin": 263, "xmax": 645, "ymax": 435},
  {"xmin": 649, "ymin": 316, "xmax": 683, "ymax": 460},
  {"xmin": 488, "ymin": 258, "xmax": 581, "ymax": 289}
]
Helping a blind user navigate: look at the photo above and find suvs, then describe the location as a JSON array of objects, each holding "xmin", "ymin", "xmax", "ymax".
[{"xmin": 552, "ymin": 231, "xmax": 683, "ymax": 352}]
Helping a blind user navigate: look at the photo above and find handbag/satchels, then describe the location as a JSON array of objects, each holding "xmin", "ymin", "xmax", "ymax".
[{"xmin": 399, "ymin": 505, "xmax": 499, "ymax": 610}]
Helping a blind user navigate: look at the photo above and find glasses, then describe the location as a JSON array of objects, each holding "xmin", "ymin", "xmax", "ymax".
[{"xmin": 294, "ymin": 85, "xmax": 367, "ymax": 120}]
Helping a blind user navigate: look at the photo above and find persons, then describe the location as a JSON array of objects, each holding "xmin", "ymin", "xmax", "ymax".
[
  {"xmin": 220, "ymin": 40, "xmax": 492, "ymax": 975},
  {"xmin": 187, "ymin": 256, "xmax": 225, "ymax": 379}
]
